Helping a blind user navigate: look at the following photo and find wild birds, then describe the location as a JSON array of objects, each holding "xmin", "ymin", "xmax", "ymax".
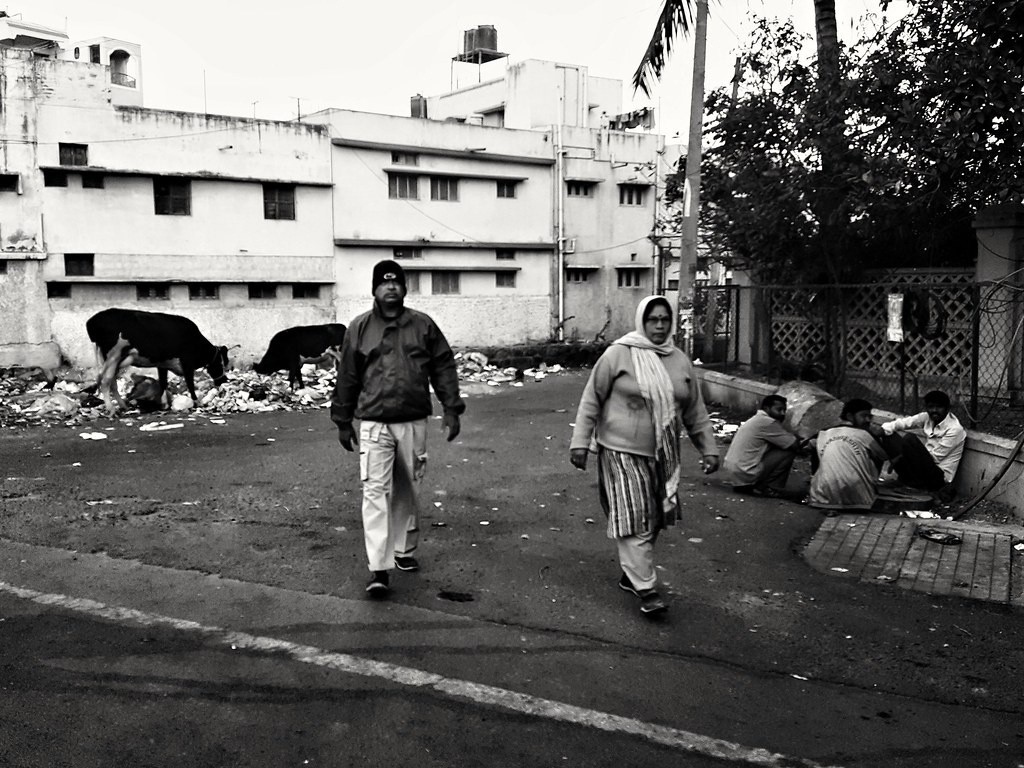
[
  {"xmin": 69, "ymin": 384, "xmax": 98, "ymax": 396},
  {"xmin": 0, "ymin": 383, "xmax": 28, "ymax": 395},
  {"xmin": 40, "ymin": 376, "xmax": 59, "ymax": 392}
]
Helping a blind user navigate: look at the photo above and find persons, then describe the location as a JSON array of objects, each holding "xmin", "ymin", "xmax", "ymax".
[
  {"xmin": 722, "ymin": 394, "xmax": 810, "ymax": 498},
  {"xmin": 568, "ymin": 295, "xmax": 721, "ymax": 613},
  {"xmin": 330, "ymin": 260, "xmax": 467, "ymax": 592},
  {"xmin": 879, "ymin": 390, "xmax": 967, "ymax": 508},
  {"xmin": 801, "ymin": 400, "xmax": 891, "ymax": 513}
]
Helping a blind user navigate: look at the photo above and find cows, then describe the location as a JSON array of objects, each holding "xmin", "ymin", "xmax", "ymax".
[
  {"xmin": 252, "ymin": 323, "xmax": 347, "ymax": 389},
  {"xmin": 86, "ymin": 307, "xmax": 231, "ymax": 414}
]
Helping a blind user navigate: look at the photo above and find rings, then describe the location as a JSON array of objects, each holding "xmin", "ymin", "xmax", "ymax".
[{"xmin": 573, "ymin": 458, "xmax": 576, "ymax": 462}]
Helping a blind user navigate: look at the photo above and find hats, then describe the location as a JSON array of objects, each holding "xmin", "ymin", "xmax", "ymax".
[{"xmin": 372, "ymin": 260, "xmax": 407, "ymax": 297}]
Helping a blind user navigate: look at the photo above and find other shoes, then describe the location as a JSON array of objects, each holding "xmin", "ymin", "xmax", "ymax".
[
  {"xmin": 894, "ymin": 486, "xmax": 921, "ymax": 495},
  {"xmin": 394, "ymin": 557, "xmax": 419, "ymax": 570},
  {"xmin": 857, "ymin": 509, "xmax": 869, "ymax": 514},
  {"xmin": 618, "ymin": 574, "xmax": 641, "ymax": 598},
  {"xmin": 884, "ymin": 478, "xmax": 905, "ymax": 488},
  {"xmin": 639, "ymin": 592, "xmax": 671, "ymax": 612},
  {"xmin": 826, "ymin": 508, "xmax": 840, "ymax": 517},
  {"xmin": 365, "ymin": 571, "xmax": 389, "ymax": 593},
  {"xmin": 753, "ymin": 485, "xmax": 792, "ymax": 498}
]
